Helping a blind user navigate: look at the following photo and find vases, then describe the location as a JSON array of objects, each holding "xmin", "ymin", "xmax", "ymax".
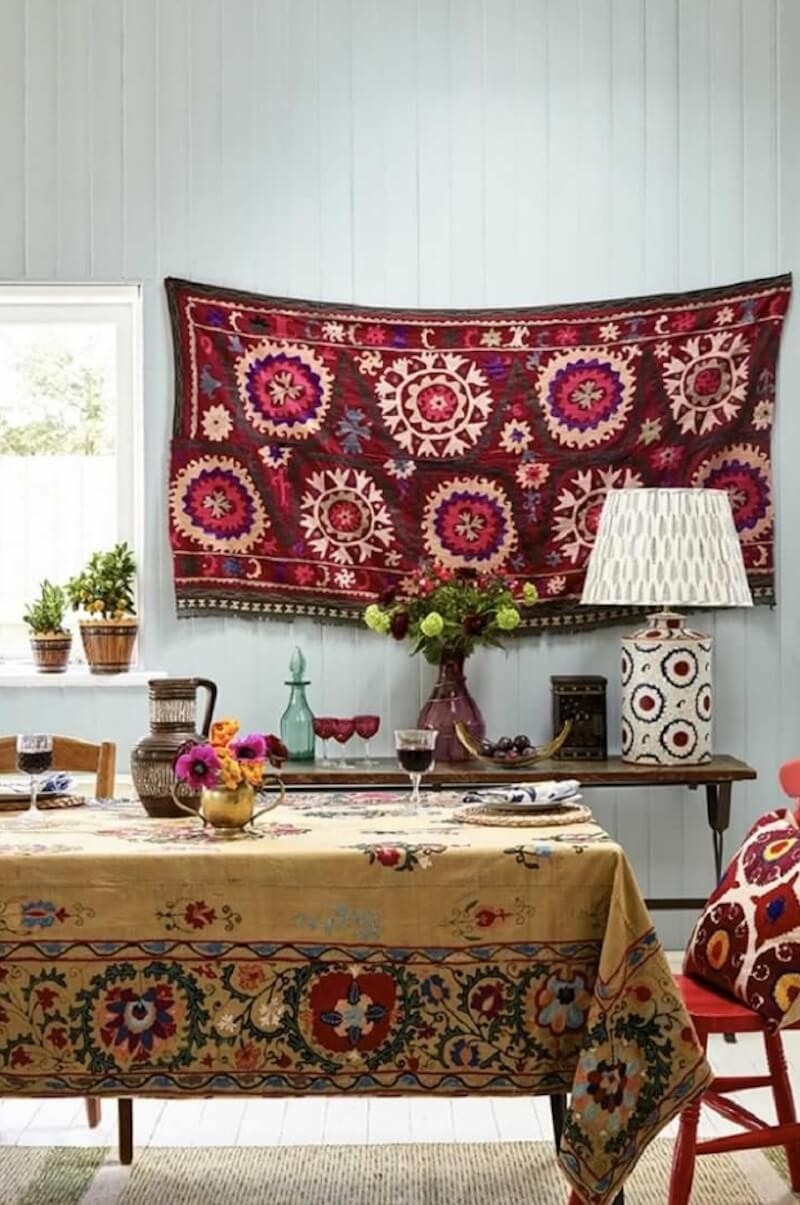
[
  {"xmin": 416, "ymin": 646, "xmax": 486, "ymax": 761},
  {"xmin": 171, "ymin": 775, "xmax": 285, "ymax": 837}
]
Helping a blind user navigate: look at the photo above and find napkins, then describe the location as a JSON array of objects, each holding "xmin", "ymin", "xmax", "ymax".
[{"xmin": 461, "ymin": 779, "xmax": 582, "ymax": 804}]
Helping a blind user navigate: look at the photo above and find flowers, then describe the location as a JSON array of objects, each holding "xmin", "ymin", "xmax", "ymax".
[
  {"xmin": 171, "ymin": 718, "xmax": 288, "ymax": 790},
  {"xmin": 358, "ymin": 558, "xmax": 538, "ymax": 665}
]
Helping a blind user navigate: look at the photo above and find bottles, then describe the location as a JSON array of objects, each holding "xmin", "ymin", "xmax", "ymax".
[
  {"xmin": 130, "ymin": 675, "xmax": 217, "ymax": 818},
  {"xmin": 280, "ymin": 644, "xmax": 316, "ymax": 764}
]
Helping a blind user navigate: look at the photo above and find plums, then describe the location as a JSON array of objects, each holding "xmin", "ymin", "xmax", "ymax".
[{"xmin": 478, "ymin": 735, "xmax": 535, "ymax": 760}]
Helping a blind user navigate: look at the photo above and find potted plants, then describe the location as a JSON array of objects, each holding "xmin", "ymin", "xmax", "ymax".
[
  {"xmin": 61, "ymin": 541, "xmax": 138, "ymax": 676},
  {"xmin": 23, "ymin": 578, "xmax": 73, "ymax": 674}
]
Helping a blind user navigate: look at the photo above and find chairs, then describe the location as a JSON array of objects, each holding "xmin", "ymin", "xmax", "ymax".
[
  {"xmin": 0, "ymin": 735, "xmax": 117, "ymax": 1129},
  {"xmin": 669, "ymin": 757, "xmax": 800, "ymax": 1205}
]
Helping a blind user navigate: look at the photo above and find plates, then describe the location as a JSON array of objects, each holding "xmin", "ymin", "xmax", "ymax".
[{"xmin": 452, "ymin": 720, "xmax": 574, "ymax": 770}]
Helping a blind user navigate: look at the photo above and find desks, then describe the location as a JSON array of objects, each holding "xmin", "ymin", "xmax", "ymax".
[{"xmin": 1, "ymin": 756, "xmax": 756, "ymax": 1205}]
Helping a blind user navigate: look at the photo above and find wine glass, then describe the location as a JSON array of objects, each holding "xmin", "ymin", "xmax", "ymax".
[
  {"xmin": 393, "ymin": 728, "xmax": 439, "ymax": 820},
  {"xmin": 14, "ymin": 732, "xmax": 54, "ymax": 824},
  {"xmin": 312, "ymin": 715, "xmax": 380, "ymax": 771}
]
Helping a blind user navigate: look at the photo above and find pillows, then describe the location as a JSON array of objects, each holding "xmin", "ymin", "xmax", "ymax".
[{"xmin": 680, "ymin": 807, "xmax": 800, "ymax": 1037}]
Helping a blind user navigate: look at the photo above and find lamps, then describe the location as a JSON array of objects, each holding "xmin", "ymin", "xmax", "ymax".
[{"xmin": 577, "ymin": 487, "xmax": 755, "ymax": 767}]
